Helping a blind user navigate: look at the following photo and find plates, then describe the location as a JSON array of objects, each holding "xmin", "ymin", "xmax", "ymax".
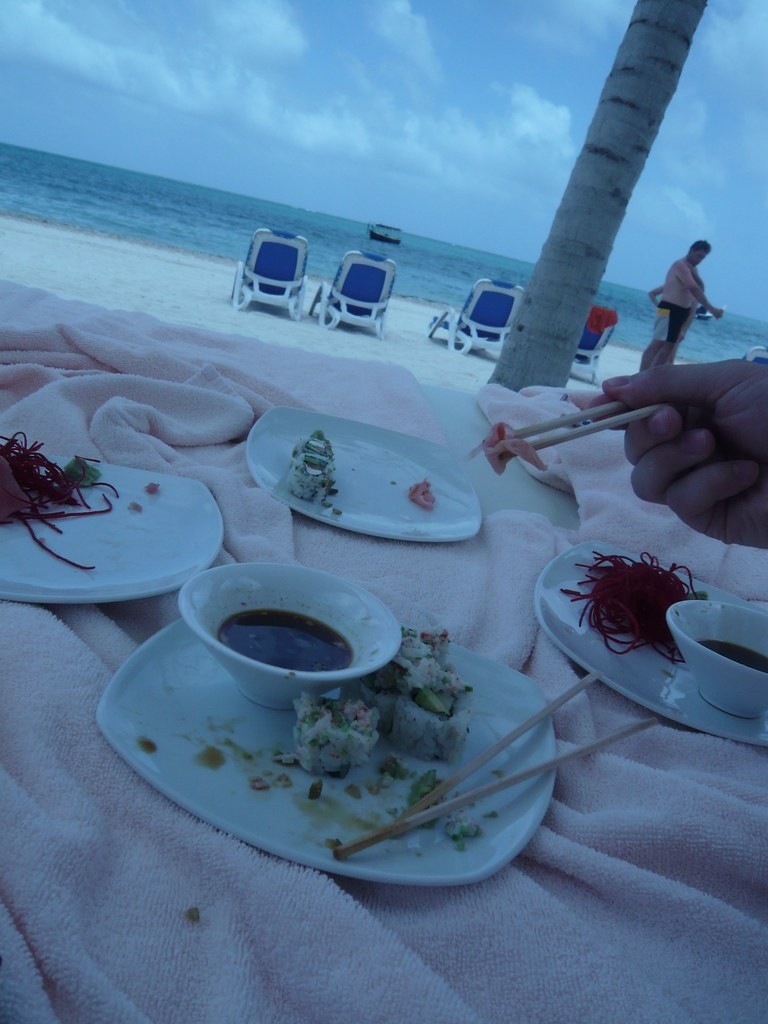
[
  {"xmin": 245, "ymin": 405, "xmax": 482, "ymax": 542},
  {"xmin": 534, "ymin": 541, "xmax": 768, "ymax": 743},
  {"xmin": 0, "ymin": 453, "xmax": 223, "ymax": 603},
  {"xmin": 96, "ymin": 617, "xmax": 557, "ymax": 886}
]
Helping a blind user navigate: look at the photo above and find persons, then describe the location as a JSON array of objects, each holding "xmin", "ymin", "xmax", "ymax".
[
  {"xmin": 585, "ymin": 360, "xmax": 768, "ymax": 550},
  {"xmin": 636, "ymin": 240, "xmax": 724, "ymax": 374}
]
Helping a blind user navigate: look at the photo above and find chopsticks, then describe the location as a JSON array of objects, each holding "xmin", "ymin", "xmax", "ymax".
[
  {"xmin": 499, "ymin": 400, "xmax": 673, "ymax": 460},
  {"xmin": 333, "ymin": 670, "xmax": 657, "ymax": 862}
]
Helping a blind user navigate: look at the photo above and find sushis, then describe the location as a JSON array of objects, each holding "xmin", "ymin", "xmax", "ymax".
[
  {"xmin": 292, "ymin": 627, "xmax": 474, "ymax": 777},
  {"xmin": 288, "ymin": 428, "xmax": 336, "ymax": 502}
]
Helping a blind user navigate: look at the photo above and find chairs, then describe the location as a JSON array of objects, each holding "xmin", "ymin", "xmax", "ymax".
[{"xmin": 230, "ymin": 227, "xmax": 618, "ymax": 390}]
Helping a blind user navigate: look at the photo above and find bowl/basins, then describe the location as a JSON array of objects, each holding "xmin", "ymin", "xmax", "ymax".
[
  {"xmin": 666, "ymin": 599, "xmax": 768, "ymax": 718},
  {"xmin": 178, "ymin": 562, "xmax": 402, "ymax": 710}
]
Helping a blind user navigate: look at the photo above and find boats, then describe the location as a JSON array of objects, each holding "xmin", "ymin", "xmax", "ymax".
[{"xmin": 367, "ymin": 219, "xmax": 402, "ymax": 244}]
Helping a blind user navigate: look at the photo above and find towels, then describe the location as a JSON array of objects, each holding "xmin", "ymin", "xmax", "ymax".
[{"xmin": 1, "ymin": 278, "xmax": 767, "ymax": 1024}]
